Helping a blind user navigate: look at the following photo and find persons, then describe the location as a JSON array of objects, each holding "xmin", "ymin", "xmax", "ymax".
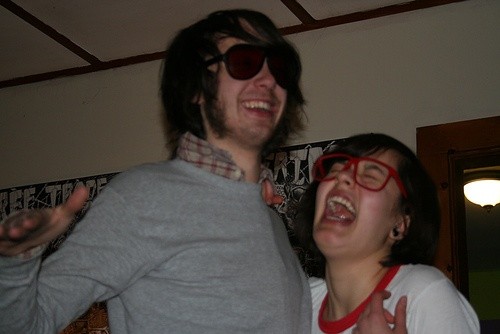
[
  {"xmin": 262, "ymin": 133, "xmax": 481, "ymax": 333},
  {"xmin": 0, "ymin": 9, "xmax": 310, "ymax": 333}
]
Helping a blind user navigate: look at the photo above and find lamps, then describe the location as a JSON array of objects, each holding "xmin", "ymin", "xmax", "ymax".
[{"xmin": 464, "ymin": 177, "xmax": 500, "ymax": 213}]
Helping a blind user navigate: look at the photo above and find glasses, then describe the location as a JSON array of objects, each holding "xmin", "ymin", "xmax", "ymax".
[
  {"xmin": 313, "ymin": 152, "xmax": 409, "ymax": 203},
  {"xmin": 203, "ymin": 44, "xmax": 303, "ymax": 89}
]
